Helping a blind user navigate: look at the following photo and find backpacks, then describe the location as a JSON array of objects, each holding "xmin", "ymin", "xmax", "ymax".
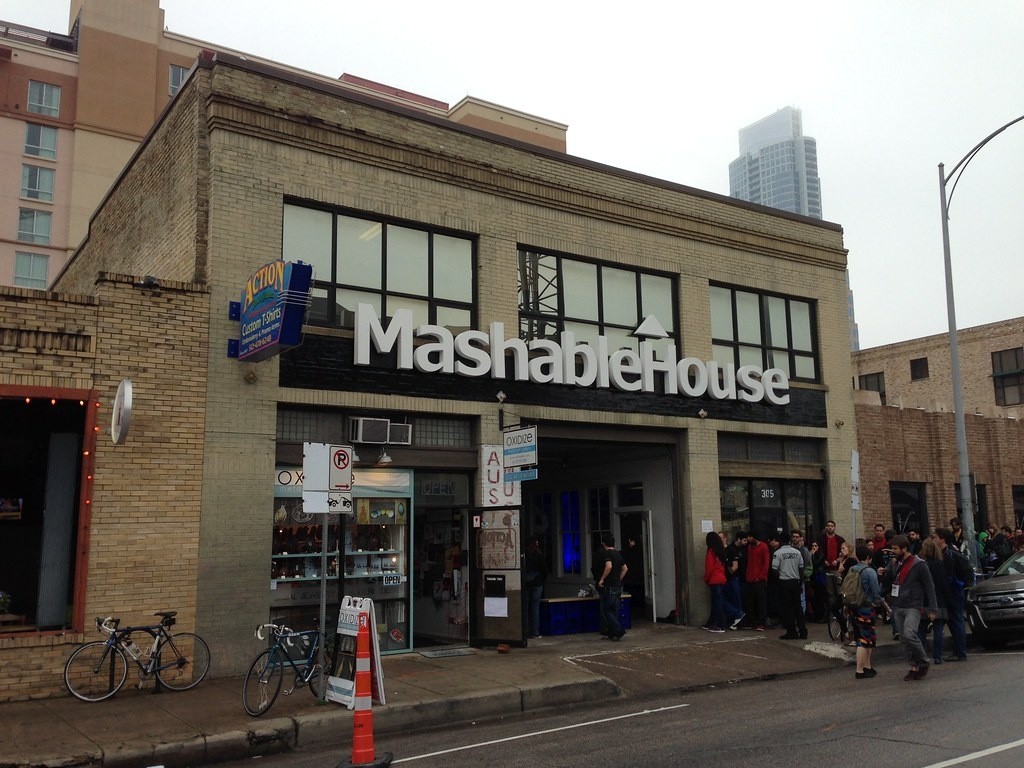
[{"xmin": 841, "ymin": 566, "xmax": 870, "ymax": 606}]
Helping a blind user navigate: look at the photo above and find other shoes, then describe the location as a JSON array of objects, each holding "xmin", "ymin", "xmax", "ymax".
[
  {"xmin": 864, "ymin": 667, "xmax": 878, "ymax": 675},
  {"xmin": 779, "ymin": 633, "xmax": 798, "ymax": 639},
  {"xmin": 529, "ymin": 634, "xmax": 543, "ymax": 639},
  {"xmin": 927, "ymin": 623, "xmax": 934, "ymax": 634},
  {"xmin": 613, "ymin": 630, "xmax": 625, "ymax": 641},
  {"xmin": 892, "ymin": 629, "xmax": 900, "ymax": 640},
  {"xmin": 855, "ymin": 671, "xmax": 873, "ymax": 679},
  {"xmin": 919, "ymin": 660, "xmax": 932, "ymax": 676},
  {"xmin": 903, "ymin": 670, "xmax": 921, "ymax": 681},
  {"xmin": 602, "ymin": 633, "xmax": 615, "ymax": 640},
  {"xmin": 810, "ymin": 611, "xmax": 830, "ymax": 623},
  {"xmin": 799, "ymin": 631, "xmax": 807, "ymax": 640},
  {"xmin": 731, "ymin": 613, "xmax": 787, "ymax": 630},
  {"xmin": 943, "ymin": 655, "xmax": 967, "ymax": 662}
]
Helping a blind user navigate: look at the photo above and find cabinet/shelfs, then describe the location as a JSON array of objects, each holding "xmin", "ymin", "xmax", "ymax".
[{"xmin": 271, "ymin": 522, "xmax": 404, "ymax": 584}]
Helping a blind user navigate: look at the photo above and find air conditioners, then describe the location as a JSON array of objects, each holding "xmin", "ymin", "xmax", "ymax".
[
  {"xmin": 388, "ymin": 424, "xmax": 412, "ymax": 445},
  {"xmin": 348, "ymin": 416, "xmax": 390, "ymax": 444}
]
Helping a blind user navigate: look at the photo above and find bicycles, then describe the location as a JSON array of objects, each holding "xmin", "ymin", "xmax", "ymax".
[
  {"xmin": 64, "ymin": 610, "xmax": 211, "ymax": 704},
  {"xmin": 242, "ymin": 613, "xmax": 336, "ymax": 717},
  {"xmin": 824, "ymin": 571, "xmax": 853, "ymax": 642}
]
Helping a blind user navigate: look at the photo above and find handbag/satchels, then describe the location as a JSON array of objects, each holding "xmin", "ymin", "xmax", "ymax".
[
  {"xmin": 986, "ymin": 550, "xmax": 998, "ymax": 561},
  {"xmin": 995, "ymin": 541, "xmax": 1011, "ymax": 557}
]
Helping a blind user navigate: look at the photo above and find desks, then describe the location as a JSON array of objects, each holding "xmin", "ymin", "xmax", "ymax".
[
  {"xmin": 412, "ymin": 590, "xmax": 468, "ymax": 640},
  {"xmin": 537, "ymin": 593, "xmax": 632, "ymax": 635}
]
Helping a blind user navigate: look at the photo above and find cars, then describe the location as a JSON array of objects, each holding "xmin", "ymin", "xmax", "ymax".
[{"xmin": 965, "ymin": 545, "xmax": 1024, "ymax": 649}]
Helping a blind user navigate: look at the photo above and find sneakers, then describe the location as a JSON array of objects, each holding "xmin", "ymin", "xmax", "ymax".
[
  {"xmin": 701, "ymin": 624, "xmax": 710, "ymax": 630},
  {"xmin": 708, "ymin": 626, "xmax": 726, "ymax": 632}
]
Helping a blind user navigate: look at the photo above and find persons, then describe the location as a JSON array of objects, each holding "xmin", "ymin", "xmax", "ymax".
[
  {"xmin": 599, "ymin": 534, "xmax": 636, "ymax": 640},
  {"xmin": 521, "ymin": 536, "xmax": 547, "ymax": 639},
  {"xmin": 703, "ymin": 516, "xmax": 1024, "ymax": 680}
]
